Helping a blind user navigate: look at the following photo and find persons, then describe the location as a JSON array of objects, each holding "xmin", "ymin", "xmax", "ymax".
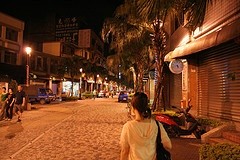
[
  {"xmin": 93, "ymin": 90, "xmax": 97, "ymax": 99},
  {"xmin": 14, "ymin": 85, "xmax": 26, "ymax": 122},
  {"xmin": 120, "ymin": 93, "xmax": 172, "ymax": 160},
  {"xmin": 5, "ymin": 88, "xmax": 15, "ymax": 120},
  {"xmin": 0, "ymin": 87, "xmax": 7, "ymax": 119}
]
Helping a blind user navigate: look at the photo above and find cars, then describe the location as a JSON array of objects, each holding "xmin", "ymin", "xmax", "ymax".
[
  {"xmin": 118, "ymin": 90, "xmax": 135, "ymax": 103},
  {"xmin": 81, "ymin": 89, "xmax": 116, "ymax": 99}
]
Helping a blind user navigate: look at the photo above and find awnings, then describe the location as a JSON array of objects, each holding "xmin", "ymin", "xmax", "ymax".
[
  {"xmin": 30, "ymin": 71, "xmax": 66, "ymax": 81},
  {"xmin": 170, "ymin": 19, "xmax": 240, "ymax": 59}
]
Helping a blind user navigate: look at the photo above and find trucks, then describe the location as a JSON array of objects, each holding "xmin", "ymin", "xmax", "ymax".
[{"xmin": 22, "ymin": 85, "xmax": 57, "ymax": 104}]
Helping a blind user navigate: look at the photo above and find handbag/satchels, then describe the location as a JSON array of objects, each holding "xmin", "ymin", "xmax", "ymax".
[{"xmin": 155, "ymin": 120, "xmax": 171, "ymax": 160}]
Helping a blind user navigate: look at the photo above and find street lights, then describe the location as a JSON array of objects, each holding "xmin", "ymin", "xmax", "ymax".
[
  {"xmin": 23, "ymin": 46, "xmax": 32, "ymax": 111},
  {"xmin": 78, "ymin": 67, "xmax": 83, "ymax": 100}
]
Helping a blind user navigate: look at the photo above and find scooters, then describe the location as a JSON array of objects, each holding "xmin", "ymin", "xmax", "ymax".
[{"xmin": 155, "ymin": 98, "xmax": 207, "ymax": 141}]
